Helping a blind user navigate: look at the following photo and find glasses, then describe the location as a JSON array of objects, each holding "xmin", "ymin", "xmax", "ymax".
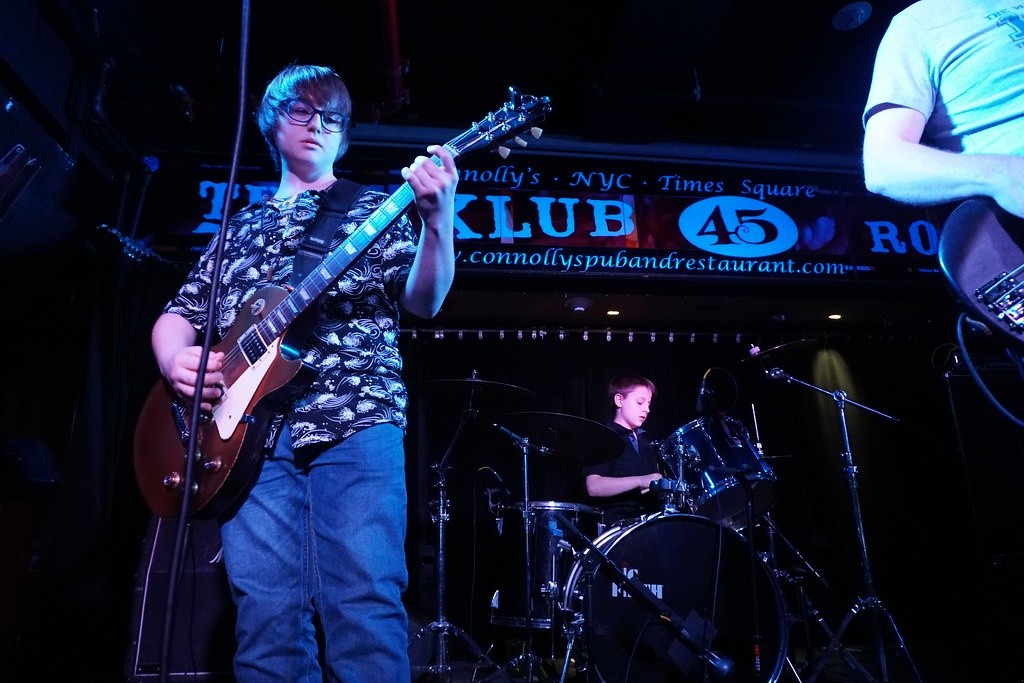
[{"xmin": 274, "ymin": 99, "xmax": 349, "ymax": 133}]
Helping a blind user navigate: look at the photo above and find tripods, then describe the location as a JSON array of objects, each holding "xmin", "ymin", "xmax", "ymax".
[
  {"xmin": 407, "ymin": 385, "xmax": 563, "ymax": 683},
  {"xmin": 750, "ymin": 367, "xmax": 925, "ymax": 683}
]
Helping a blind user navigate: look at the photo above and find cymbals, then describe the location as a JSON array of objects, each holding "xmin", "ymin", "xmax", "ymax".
[
  {"xmin": 418, "ymin": 377, "xmax": 535, "ymax": 397},
  {"xmin": 490, "ymin": 410, "xmax": 628, "ymax": 467},
  {"xmin": 704, "ymin": 337, "xmax": 819, "ymax": 383}
]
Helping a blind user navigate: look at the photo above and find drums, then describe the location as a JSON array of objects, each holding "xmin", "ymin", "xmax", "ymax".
[
  {"xmin": 562, "ymin": 513, "xmax": 787, "ymax": 683},
  {"xmin": 655, "ymin": 413, "xmax": 779, "ymax": 530},
  {"xmin": 487, "ymin": 499, "xmax": 606, "ymax": 631}
]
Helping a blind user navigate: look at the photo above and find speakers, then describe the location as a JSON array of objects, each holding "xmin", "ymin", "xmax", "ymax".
[{"xmin": 124, "ymin": 511, "xmax": 239, "ymax": 683}]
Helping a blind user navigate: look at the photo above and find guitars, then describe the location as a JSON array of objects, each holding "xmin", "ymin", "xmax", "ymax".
[
  {"xmin": 937, "ymin": 197, "xmax": 1023, "ymax": 344},
  {"xmin": 132, "ymin": 87, "xmax": 553, "ymax": 529}
]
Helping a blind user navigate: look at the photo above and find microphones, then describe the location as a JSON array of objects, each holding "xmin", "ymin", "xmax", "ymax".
[
  {"xmin": 696, "ymin": 378, "xmax": 707, "ymax": 412},
  {"xmin": 660, "ymin": 614, "xmax": 736, "ymax": 679}
]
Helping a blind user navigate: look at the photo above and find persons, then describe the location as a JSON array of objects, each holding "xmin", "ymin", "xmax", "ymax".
[
  {"xmin": 151, "ymin": 67, "xmax": 460, "ymax": 681},
  {"xmin": 584, "ymin": 369, "xmax": 662, "ymax": 528},
  {"xmin": 860, "ymin": 0, "xmax": 1023, "ymax": 223}
]
